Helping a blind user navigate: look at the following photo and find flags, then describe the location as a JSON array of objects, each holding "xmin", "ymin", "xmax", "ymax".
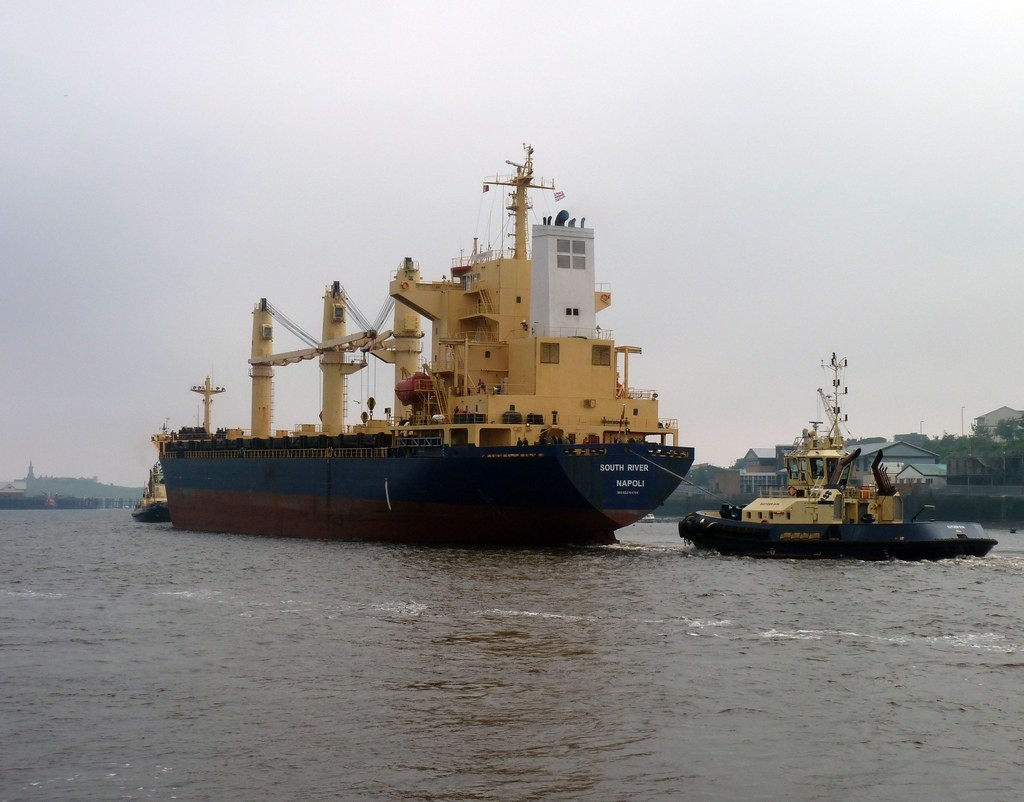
[
  {"xmin": 483, "ymin": 185, "xmax": 489, "ymax": 193},
  {"xmin": 554, "ymin": 191, "xmax": 565, "ymax": 201}
]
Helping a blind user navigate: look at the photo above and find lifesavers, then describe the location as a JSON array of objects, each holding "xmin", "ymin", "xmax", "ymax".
[
  {"xmin": 601, "ymin": 294, "xmax": 607, "ymax": 301},
  {"xmin": 402, "ymin": 282, "xmax": 409, "ymax": 289},
  {"xmin": 788, "ymin": 487, "xmax": 796, "ymax": 496}
]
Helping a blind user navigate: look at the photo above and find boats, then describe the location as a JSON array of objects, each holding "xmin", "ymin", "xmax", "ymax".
[
  {"xmin": 145, "ymin": 147, "xmax": 698, "ymax": 540},
  {"xmin": 132, "ymin": 462, "xmax": 169, "ymax": 522},
  {"xmin": 673, "ymin": 351, "xmax": 1000, "ymax": 559}
]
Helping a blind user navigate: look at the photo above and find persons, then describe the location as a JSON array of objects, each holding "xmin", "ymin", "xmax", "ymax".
[
  {"xmin": 477, "ymin": 378, "xmax": 487, "ymax": 393},
  {"xmin": 583, "ymin": 437, "xmax": 587, "ymax": 444},
  {"xmin": 517, "ymin": 437, "xmax": 522, "ymax": 446},
  {"xmin": 522, "ymin": 437, "xmax": 528, "ymax": 446},
  {"xmin": 454, "ymin": 406, "xmax": 459, "ymax": 413},
  {"xmin": 665, "ymin": 423, "xmax": 669, "ymax": 429}
]
